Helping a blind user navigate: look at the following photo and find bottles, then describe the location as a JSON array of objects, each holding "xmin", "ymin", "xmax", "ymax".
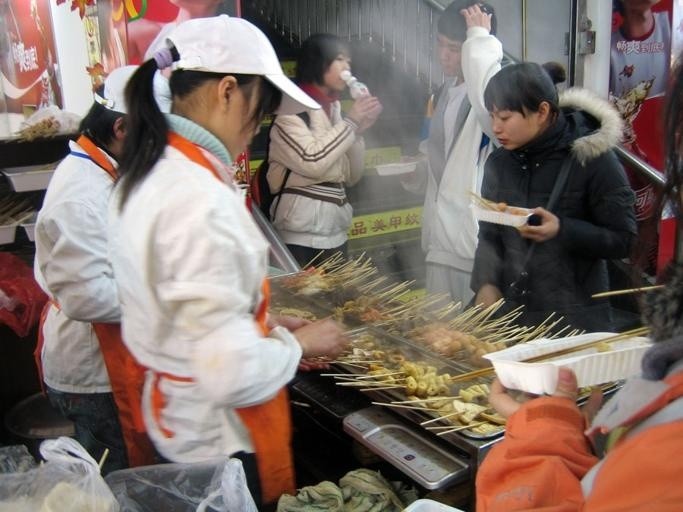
[
  {"xmin": 339, "ymin": 70, "xmax": 371, "ymax": 100},
  {"xmin": 622, "ymin": 126, "xmax": 659, "ymax": 276}
]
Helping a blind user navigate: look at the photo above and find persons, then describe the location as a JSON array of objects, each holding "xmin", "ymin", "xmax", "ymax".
[
  {"xmin": 102, "ymin": 11, "xmax": 349, "ymax": 511},
  {"xmin": 462, "ymin": 61, "xmax": 640, "ymax": 349},
  {"xmin": 608, "ymin": 0, "xmax": 675, "ymax": 275},
  {"xmin": 248, "ymin": 33, "xmax": 383, "ymax": 273},
  {"xmin": 415, "ymin": 0, "xmax": 506, "ymax": 314},
  {"xmin": 469, "ymin": 51, "xmax": 681, "ymax": 510},
  {"xmin": 31, "ymin": 62, "xmax": 172, "ymax": 478},
  {"xmin": 112, "ymin": 1, "xmax": 237, "ymax": 61}
]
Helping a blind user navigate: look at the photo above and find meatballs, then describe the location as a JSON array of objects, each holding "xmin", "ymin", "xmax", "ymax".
[
  {"xmin": 498, "ymin": 203, "xmax": 528, "ymax": 217},
  {"xmin": 423, "ymin": 326, "xmax": 506, "ymax": 367}
]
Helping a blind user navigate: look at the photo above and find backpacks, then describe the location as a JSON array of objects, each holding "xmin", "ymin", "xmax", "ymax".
[{"xmin": 250, "ymin": 111, "xmax": 310, "ymax": 219}]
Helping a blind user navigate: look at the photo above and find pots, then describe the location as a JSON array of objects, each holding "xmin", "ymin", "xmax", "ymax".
[{"xmin": 5, "ymin": 387, "xmax": 77, "ymax": 462}]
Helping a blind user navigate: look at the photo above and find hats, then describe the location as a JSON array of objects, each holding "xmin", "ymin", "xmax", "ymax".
[
  {"xmin": 93, "ymin": 63, "xmax": 175, "ymax": 117},
  {"xmin": 165, "ymin": 13, "xmax": 321, "ymax": 109}
]
